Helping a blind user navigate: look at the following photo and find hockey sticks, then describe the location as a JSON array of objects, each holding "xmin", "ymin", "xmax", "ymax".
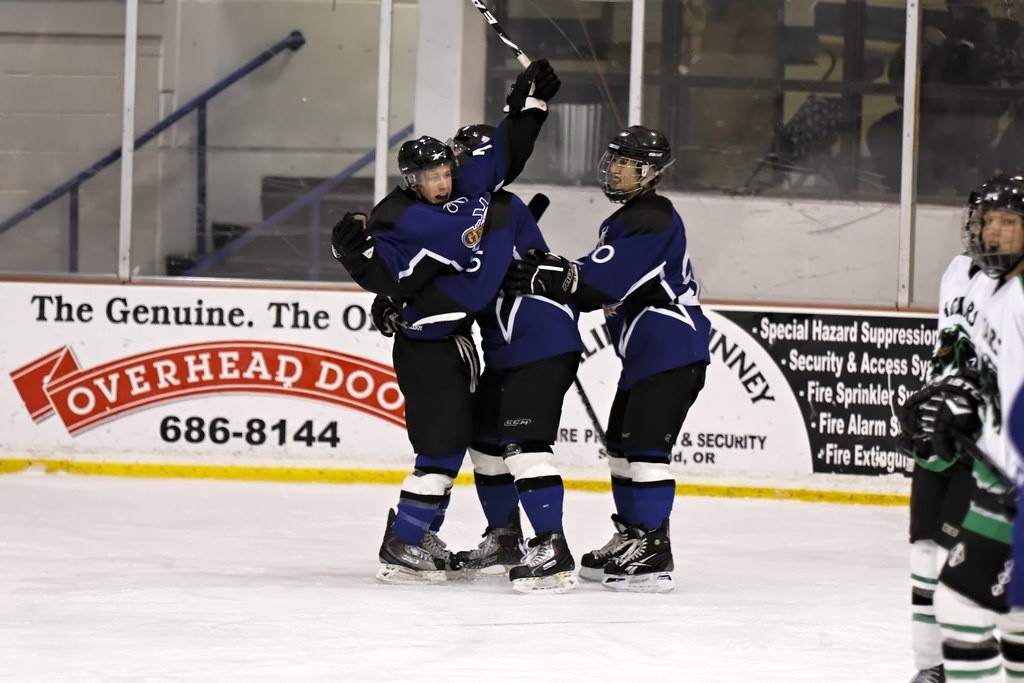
[
  {"xmin": 471, "ymin": 0, "xmax": 532, "ymax": 68},
  {"xmin": 574, "ymin": 375, "xmax": 608, "ymax": 445},
  {"xmin": 960, "ymin": 434, "xmax": 1014, "ymax": 491}
]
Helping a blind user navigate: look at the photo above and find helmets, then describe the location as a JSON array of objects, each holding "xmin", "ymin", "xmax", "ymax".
[
  {"xmin": 454, "ymin": 123, "xmax": 501, "ymax": 156},
  {"xmin": 897, "ymin": 379, "xmax": 980, "ymax": 468},
  {"xmin": 596, "ymin": 125, "xmax": 673, "ymax": 201},
  {"xmin": 398, "ymin": 137, "xmax": 456, "ymax": 173},
  {"xmin": 962, "ymin": 172, "xmax": 1024, "ymax": 276}
]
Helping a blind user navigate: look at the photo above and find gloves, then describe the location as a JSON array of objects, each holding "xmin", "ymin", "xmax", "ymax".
[
  {"xmin": 371, "ymin": 297, "xmax": 394, "ymax": 337},
  {"xmin": 517, "ymin": 58, "xmax": 562, "ymax": 97},
  {"xmin": 531, "ymin": 253, "xmax": 571, "ymax": 296},
  {"xmin": 331, "ymin": 212, "xmax": 372, "ymax": 254}
]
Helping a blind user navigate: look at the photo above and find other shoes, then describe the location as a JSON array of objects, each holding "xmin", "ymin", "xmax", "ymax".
[{"xmin": 913, "ymin": 667, "xmax": 941, "ymax": 681}]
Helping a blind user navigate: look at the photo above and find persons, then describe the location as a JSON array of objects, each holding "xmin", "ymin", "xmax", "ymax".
[
  {"xmin": 331, "ymin": 57, "xmax": 561, "ymax": 583},
  {"xmin": 500, "ymin": 126, "xmax": 711, "ymax": 593},
  {"xmin": 370, "ymin": 124, "xmax": 582, "ymax": 594},
  {"xmin": 895, "ymin": 175, "xmax": 1024, "ymax": 683},
  {"xmin": 866, "ymin": 0, "xmax": 1002, "ymax": 205}
]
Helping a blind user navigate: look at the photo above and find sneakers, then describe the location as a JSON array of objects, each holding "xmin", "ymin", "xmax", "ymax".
[
  {"xmin": 457, "ymin": 530, "xmax": 575, "ymax": 582},
  {"xmin": 580, "ymin": 515, "xmax": 674, "ymax": 581},
  {"xmin": 378, "ymin": 509, "xmax": 458, "ymax": 573}
]
